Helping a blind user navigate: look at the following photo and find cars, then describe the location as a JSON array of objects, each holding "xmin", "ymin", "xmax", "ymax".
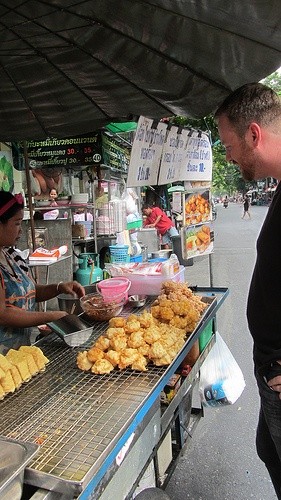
[{"xmin": 214, "ymin": 198, "xmax": 219, "ymax": 204}]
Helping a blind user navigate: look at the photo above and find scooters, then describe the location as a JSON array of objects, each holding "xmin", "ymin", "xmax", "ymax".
[{"xmin": 223, "ymin": 201, "xmax": 229, "ymax": 208}]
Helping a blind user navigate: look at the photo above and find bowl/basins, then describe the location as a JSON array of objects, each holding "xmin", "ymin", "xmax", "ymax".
[
  {"xmin": 128, "ymin": 294, "xmax": 148, "ymax": 307},
  {"xmin": 79, "ymin": 292, "xmax": 127, "ymax": 321}
]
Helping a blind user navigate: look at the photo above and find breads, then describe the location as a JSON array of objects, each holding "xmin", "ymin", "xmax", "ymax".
[
  {"xmin": 0, "ymin": 345, "xmax": 49, "ymax": 400},
  {"xmin": 196, "ymin": 225, "xmax": 210, "ymax": 253}
]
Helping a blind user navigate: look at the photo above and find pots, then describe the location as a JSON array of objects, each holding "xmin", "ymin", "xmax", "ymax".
[{"xmin": 57, "ymin": 296, "xmax": 82, "ymax": 315}]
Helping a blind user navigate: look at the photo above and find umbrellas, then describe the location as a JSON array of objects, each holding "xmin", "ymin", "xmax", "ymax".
[
  {"xmin": 0, "ymin": 0, "xmax": 281, "ymax": 145},
  {"xmin": 168, "ymin": 185, "xmax": 184, "ymax": 194}
]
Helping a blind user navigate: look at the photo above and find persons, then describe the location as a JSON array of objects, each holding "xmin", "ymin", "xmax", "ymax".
[
  {"xmin": 48, "ymin": 188, "xmax": 58, "ymax": 201},
  {"xmin": 141, "ymin": 202, "xmax": 182, "ymax": 265},
  {"xmin": 223, "ymin": 196, "xmax": 229, "ymax": 207},
  {"xmin": 214, "ymin": 83, "xmax": 280, "ymax": 500},
  {"xmin": 0, "ymin": 188, "xmax": 85, "ymax": 356},
  {"xmin": 95, "ymin": 186, "xmax": 108, "ymax": 203},
  {"xmin": 252, "ymin": 191, "xmax": 274, "ymax": 206},
  {"xmin": 241, "ymin": 195, "xmax": 251, "ymax": 219}
]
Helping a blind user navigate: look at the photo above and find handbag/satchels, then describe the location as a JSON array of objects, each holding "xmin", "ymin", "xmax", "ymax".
[{"xmin": 196, "ymin": 332, "xmax": 246, "ymax": 406}]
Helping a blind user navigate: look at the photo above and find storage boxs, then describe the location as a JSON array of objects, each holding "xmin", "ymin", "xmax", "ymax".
[{"xmin": 111, "ymin": 265, "xmax": 185, "ymax": 294}]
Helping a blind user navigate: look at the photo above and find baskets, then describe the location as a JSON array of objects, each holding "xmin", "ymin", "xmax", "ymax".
[{"xmin": 108, "ymin": 244, "xmax": 129, "ymax": 263}]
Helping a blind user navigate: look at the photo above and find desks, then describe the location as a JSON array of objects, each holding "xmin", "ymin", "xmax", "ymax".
[{"xmin": 28, "ymin": 254, "xmax": 71, "ymax": 312}]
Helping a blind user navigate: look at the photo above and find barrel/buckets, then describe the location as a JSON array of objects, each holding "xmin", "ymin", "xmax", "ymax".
[{"xmin": 95, "ymin": 277, "xmax": 131, "ymax": 305}]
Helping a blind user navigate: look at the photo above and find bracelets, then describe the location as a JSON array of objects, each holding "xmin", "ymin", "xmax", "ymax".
[{"xmin": 56, "ymin": 281, "xmax": 64, "ymax": 293}]
